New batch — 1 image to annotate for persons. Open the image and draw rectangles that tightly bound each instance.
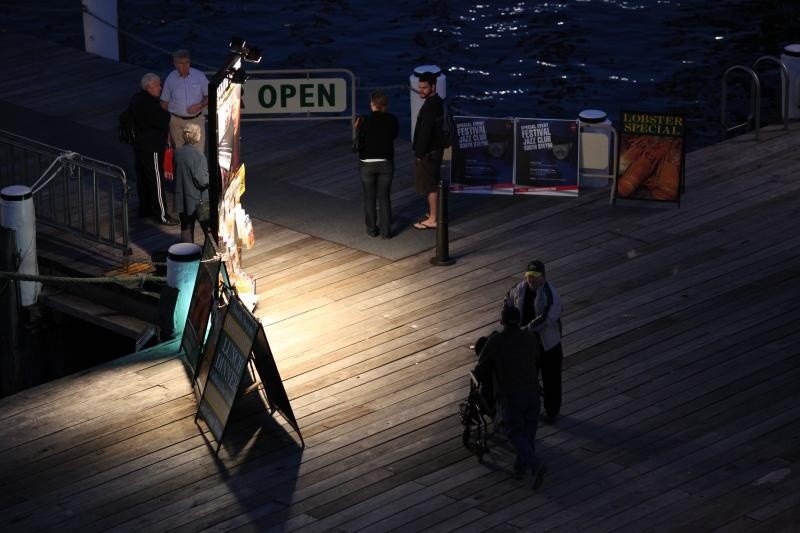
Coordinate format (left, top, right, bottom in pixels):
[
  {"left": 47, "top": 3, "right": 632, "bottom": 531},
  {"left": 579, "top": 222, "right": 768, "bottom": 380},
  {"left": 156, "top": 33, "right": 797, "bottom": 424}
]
[
  {"left": 411, "top": 71, "right": 453, "bottom": 230},
  {"left": 353, "top": 91, "right": 399, "bottom": 241},
  {"left": 471, "top": 260, "right": 565, "bottom": 452},
  {"left": 172, "top": 122, "right": 210, "bottom": 244},
  {"left": 119, "top": 49, "right": 210, "bottom": 226}
]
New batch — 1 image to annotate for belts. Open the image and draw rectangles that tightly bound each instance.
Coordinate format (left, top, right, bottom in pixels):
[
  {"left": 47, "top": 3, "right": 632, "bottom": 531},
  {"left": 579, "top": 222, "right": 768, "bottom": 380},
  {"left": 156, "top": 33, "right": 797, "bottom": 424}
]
[{"left": 171, "top": 111, "right": 203, "bottom": 120}]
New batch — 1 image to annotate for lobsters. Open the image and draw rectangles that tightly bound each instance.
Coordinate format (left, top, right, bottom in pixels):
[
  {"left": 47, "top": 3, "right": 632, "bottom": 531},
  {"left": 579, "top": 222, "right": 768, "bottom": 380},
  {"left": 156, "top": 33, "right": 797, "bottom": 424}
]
[{"left": 617, "top": 136, "right": 682, "bottom": 201}]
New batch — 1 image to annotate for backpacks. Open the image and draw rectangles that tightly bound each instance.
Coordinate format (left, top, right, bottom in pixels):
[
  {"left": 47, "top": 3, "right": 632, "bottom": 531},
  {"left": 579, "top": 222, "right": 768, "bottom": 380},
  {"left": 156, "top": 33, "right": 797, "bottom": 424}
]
[{"left": 431, "top": 102, "right": 456, "bottom": 149}]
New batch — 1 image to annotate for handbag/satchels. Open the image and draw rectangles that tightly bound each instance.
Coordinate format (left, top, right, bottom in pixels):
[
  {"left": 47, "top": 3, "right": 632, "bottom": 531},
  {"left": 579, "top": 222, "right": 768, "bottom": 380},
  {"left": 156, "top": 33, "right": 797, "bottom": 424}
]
[
  {"left": 117, "top": 100, "right": 135, "bottom": 145},
  {"left": 352, "top": 118, "right": 361, "bottom": 153},
  {"left": 197, "top": 201, "right": 210, "bottom": 222}
]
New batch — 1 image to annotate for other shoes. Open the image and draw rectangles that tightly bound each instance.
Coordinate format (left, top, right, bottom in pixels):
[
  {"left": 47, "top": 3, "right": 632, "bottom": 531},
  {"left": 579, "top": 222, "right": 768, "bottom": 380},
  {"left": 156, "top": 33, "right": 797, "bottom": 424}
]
[
  {"left": 512, "top": 478, "right": 526, "bottom": 488},
  {"left": 543, "top": 412, "right": 558, "bottom": 424},
  {"left": 529, "top": 459, "right": 547, "bottom": 489},
  {"left": 161, "top": 218, "right": 180, "bottom": 226}
]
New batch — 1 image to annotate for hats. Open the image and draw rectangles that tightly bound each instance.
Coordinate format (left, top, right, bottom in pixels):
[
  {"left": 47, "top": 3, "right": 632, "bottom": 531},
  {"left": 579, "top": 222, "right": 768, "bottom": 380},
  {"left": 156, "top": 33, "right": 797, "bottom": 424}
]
[{"left": 524, "top": 260, "right": 545, "bottom": 278}]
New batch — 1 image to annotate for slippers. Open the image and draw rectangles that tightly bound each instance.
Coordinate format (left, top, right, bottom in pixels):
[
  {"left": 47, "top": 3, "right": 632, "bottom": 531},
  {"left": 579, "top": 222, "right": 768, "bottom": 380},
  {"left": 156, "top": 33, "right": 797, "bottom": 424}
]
[
  {"left": 413, "top": 222, "right": 437, "bottom": 230},
  {"left": 424, "top": 212, "right": 430, "bottom": 218}
]
